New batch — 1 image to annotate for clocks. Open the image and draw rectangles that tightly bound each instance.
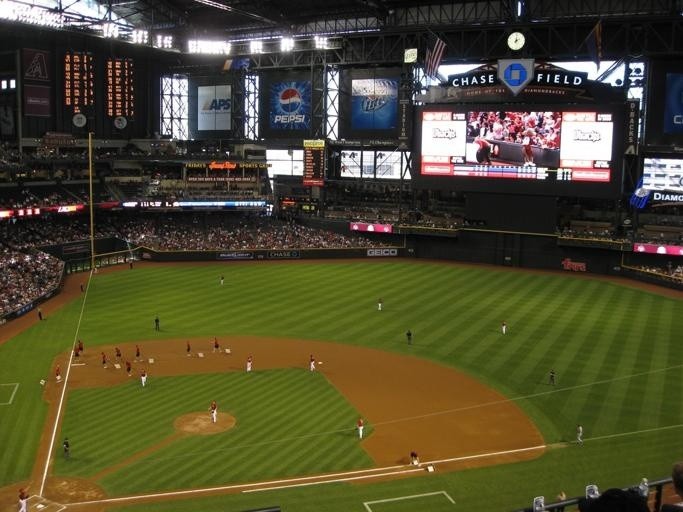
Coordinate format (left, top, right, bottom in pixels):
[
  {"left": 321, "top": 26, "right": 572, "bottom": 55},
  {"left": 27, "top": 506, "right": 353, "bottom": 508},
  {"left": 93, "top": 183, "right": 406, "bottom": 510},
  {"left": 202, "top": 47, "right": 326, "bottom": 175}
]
[{"left": 506, "top": 31, "right": 526, "bottom": 51}]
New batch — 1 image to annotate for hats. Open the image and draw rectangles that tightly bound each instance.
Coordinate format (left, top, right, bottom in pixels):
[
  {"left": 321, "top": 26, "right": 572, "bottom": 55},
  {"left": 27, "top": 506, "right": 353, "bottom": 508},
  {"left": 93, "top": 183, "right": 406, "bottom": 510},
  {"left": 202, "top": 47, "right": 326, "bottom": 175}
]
[{"left": 579, "top": 488, "right": 649, "bottom": 512}]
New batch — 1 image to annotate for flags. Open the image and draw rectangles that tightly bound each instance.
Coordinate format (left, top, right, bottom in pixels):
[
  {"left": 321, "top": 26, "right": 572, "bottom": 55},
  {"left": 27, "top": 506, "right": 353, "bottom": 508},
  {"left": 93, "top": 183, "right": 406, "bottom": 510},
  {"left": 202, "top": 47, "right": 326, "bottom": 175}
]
[
  {"left": 585, "top": 19, "right": 603, "bottom": 71},
  {"left": 425, "top": 29, "right": 447, "bottom": 81}
]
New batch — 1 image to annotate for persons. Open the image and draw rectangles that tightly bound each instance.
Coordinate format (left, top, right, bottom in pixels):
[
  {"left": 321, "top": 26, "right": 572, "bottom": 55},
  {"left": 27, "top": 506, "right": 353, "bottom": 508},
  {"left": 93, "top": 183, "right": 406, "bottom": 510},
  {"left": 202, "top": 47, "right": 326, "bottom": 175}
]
[
  {"left": 56, "top": 336, "right": 254, "bottom": 423},
  {"left": 576, "top": 424, "right": 583, "bottom": 445},
  {"left": 406, "top": 330, "right": 412, "bottom": 345},
  {"left": 500, "top": 319, "right": 506, "bottom": 334},
  {"left": 155, "top": 316, "right": 159, "bottom": 331},
  {"left": 523, "top": 462, "right": 683, "bottom": 512},
  {"left": 63, "top": 437, "right": 69, "bottom": 457},
  {"left": 549, "top": 369, "right": 556, "bottom": 385},
  {"left": 466, "top": 111, "right": 562, "bottom": 166},
  {"left": 19, "top": 488, "right": 29, "bottom": 512},
  {"left": 409, "top": 451, "right": 420, "bottom": 465},
  {"left": 357, "top": 416, "right": 363, "bottom": 439},
  {"left": 555, "top": 207, "right": 683, "bottom": 283},
  {"left": 310, "top": 354, "right": 315, "bottom": 371}
]
